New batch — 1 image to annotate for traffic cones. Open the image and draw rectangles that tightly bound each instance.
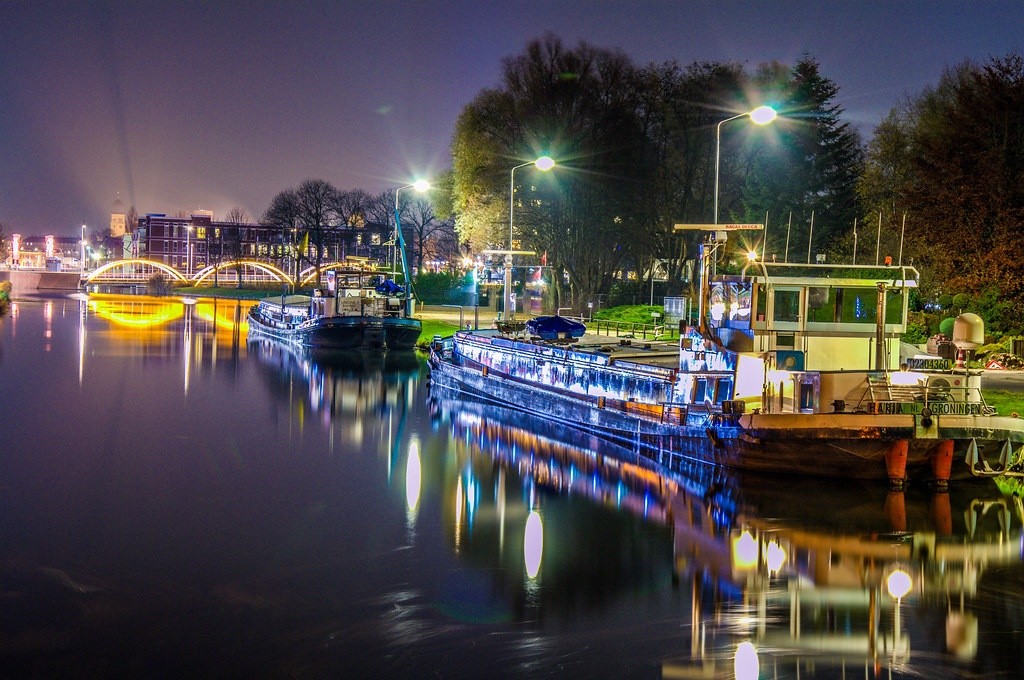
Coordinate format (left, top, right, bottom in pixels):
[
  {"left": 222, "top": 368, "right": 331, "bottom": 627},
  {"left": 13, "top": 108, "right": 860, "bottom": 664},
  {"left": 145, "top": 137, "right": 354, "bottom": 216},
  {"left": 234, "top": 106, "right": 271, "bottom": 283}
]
[{"left": 956, "top": 350, "right": 965, "bottom": 369}]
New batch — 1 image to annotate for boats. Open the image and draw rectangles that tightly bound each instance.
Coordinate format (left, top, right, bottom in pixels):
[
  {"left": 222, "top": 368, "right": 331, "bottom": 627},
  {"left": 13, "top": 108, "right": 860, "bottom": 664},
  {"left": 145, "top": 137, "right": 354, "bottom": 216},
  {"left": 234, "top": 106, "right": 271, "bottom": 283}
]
[
  {"left": 431, "top": 211, "right": 1024, "bottom": 492},
  {"left": 247, "top": 268, "right": 423, "bottom": 350}
]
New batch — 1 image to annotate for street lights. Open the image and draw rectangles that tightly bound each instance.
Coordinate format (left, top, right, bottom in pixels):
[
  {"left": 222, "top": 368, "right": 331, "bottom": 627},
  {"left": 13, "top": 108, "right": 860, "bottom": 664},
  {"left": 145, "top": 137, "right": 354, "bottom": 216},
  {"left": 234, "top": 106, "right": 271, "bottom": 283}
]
[
  {"left": 393, "top": 180, "right": 430, "bottom": 284},
  {"left": 81, "top": 224, "right": 86, "bottom": 242},
  {"left": 712, "top": 103, "right": 777, "bottom": 274},
  {"left": 186, "top": 227, "right": 193, "bottom": 278},
  {"left": 508, "top": 156, "right": 555, "bottom": 252}
]
